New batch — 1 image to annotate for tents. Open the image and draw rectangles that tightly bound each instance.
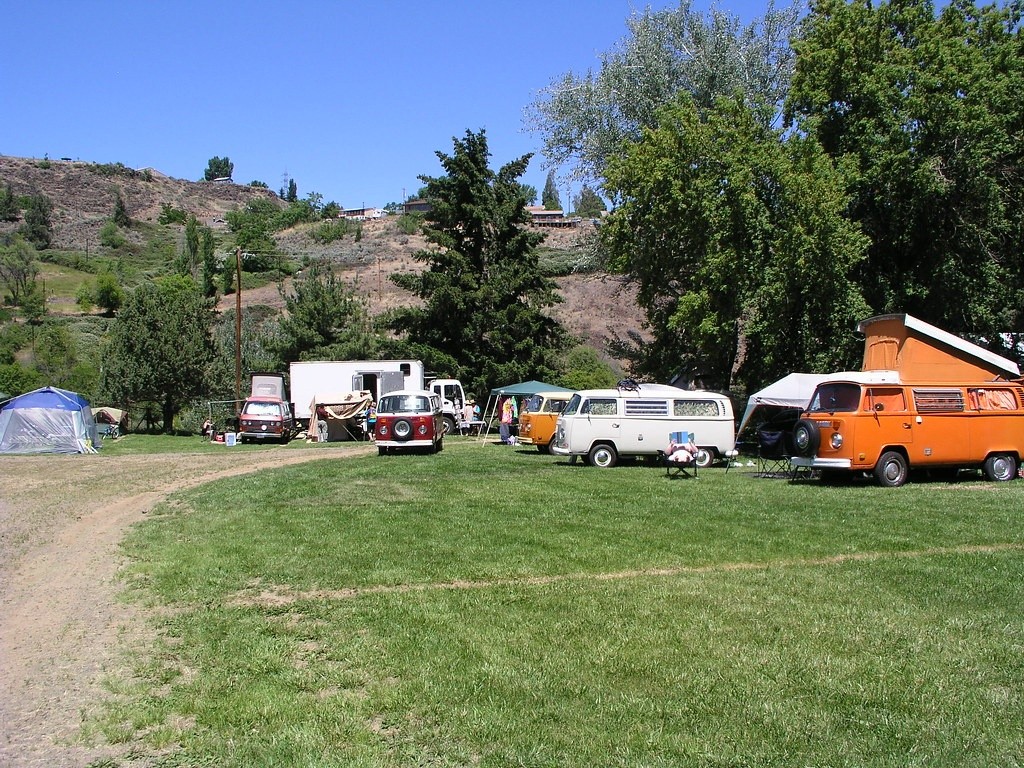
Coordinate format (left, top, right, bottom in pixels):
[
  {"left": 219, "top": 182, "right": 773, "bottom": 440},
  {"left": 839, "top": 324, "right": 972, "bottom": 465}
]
[
  {"left": 724, "top": 371, "right": 902, "bottom": 474},
  {"left": 474, "top": 379, "right": 578, "bottom": 448},
  {"left": 0, "top": 386, "right": 103, "bottom": 456}
]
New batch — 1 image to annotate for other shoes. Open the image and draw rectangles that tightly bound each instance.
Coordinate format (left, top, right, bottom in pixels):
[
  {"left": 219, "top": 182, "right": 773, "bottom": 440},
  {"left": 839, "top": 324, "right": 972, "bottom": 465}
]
[{"left": 369, "top": 438, "right": 374, "bottom": 443}]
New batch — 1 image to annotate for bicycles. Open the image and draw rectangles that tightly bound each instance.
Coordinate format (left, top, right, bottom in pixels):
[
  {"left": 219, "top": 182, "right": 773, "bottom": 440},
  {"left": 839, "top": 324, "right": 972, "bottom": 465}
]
[{"left": 102, "top": 422, "right": 120, "bottom": 440}]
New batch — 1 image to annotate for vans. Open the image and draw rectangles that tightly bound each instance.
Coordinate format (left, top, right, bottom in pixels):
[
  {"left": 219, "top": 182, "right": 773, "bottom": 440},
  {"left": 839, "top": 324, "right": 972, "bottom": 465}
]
[
  {"left": 374, "top": 390, "right": 448, "bottom": 455},
  {"left": 553, "top": 383, "right": 739, "bottom": 468},
  {"left": 517, "top": 390, "right": 572, "bottom": 454},
  {"left": 236, "top": 397, "right": 293, "bottom": 444},
  {"left": 788, "top": 311, "right": 1024, "bottom": 494}
]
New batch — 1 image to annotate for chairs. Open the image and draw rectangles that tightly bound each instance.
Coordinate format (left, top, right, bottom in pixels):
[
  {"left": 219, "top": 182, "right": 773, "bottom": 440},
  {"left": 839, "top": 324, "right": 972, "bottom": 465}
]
[
  {"left": 658, "top": 432, "right": 698, "bottom": 477},
  {"left": 753, "top": 428, "right": 792, "bottom": 478}
]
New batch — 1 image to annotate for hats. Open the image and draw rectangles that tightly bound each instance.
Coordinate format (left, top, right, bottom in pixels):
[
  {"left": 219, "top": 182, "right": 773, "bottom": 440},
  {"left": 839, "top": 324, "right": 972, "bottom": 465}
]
[
  {"left": 371, "top": 402, "right": 376, "bottom": 407},
  {"left": 464, "top": 400, "right": 472, "bottom": 404},
  {"left": 470, "top": 399, "right": 476, "bottom": 404}
]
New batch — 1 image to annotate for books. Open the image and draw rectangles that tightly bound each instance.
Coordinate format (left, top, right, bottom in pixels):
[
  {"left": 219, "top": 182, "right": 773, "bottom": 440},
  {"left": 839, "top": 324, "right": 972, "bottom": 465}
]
[{"left": 669, "top": 432, "right": 688, "bottom": 444}]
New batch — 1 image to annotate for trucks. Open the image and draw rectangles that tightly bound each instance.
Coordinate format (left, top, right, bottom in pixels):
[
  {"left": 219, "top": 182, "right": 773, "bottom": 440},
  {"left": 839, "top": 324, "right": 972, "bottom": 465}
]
[{"left": 290, "top": 359, "right": 470, "bottom": 437}]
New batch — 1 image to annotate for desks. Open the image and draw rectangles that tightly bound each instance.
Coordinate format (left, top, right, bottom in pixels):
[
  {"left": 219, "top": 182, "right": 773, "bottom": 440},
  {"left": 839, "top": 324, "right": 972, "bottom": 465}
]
[{"left": 459, "top": 420, "right": 486, "bottom": 436}]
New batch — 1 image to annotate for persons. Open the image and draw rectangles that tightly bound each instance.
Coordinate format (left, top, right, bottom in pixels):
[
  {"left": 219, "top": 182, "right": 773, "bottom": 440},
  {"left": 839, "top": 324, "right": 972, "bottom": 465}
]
[
  {"left": 665, "top": 439, "right": 699, "bottom": 463},
  {"left": 202, "top": 419, "right": 214, "bottom": 435},
  {"left": 365, "top": 402, "right": 378, "bottom": 442},
  {"left": 464, "top": 399, "right": 481, "bottom": 436},
  {"left": 316, "top": 403, "right": 328, "bottom": 442}
]
